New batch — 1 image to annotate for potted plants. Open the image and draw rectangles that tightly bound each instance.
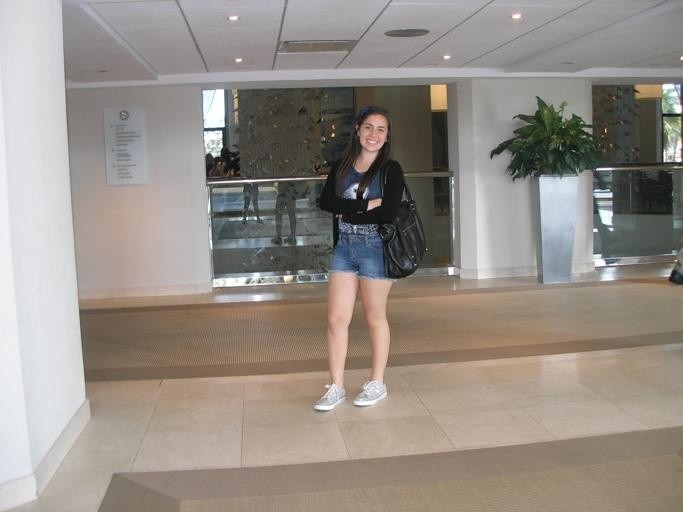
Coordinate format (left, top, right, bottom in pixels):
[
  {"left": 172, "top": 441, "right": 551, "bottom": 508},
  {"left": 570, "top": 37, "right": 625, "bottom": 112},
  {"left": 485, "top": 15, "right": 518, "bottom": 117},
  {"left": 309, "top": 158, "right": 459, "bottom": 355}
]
[{"left": 489, "top": 93, "right": 606, "bottom": 285}]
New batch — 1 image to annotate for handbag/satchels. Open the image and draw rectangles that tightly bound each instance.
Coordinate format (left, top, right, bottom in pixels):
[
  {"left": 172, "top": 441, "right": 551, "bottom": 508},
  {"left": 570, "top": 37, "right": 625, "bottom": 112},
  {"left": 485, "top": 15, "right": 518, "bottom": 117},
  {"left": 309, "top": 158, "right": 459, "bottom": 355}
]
[{"left": 375, "top": 158, "right": 427, "bottom": 279}]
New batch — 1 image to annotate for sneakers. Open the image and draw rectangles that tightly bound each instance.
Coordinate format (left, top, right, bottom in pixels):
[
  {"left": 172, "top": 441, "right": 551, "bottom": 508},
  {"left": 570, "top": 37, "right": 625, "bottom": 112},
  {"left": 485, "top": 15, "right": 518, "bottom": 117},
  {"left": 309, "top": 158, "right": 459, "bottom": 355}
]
[
  {"left": 257, "top": 218, "right": 263, "bottom": 223},
  {"left": 242, "top": 220, "right": 247, "bottom": 223},
  {"left": 284, "top": 238, "right": 296, "bottom": 245},
  {"left": 271, "top": 238, "right": 282, "bottom": 245},
  {"left": 313, "top": 382, "right": 346, "bottom": 411},
  {"left": 352, "top": 380, "right": 387, "bottom": 406}
]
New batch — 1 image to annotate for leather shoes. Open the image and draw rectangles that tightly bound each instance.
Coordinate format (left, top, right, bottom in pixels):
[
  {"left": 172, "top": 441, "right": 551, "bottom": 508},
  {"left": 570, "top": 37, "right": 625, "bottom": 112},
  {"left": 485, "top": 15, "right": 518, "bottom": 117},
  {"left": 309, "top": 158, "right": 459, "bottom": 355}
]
[{"left": 668, "top": 270, "right": 682, "bottom": 284}]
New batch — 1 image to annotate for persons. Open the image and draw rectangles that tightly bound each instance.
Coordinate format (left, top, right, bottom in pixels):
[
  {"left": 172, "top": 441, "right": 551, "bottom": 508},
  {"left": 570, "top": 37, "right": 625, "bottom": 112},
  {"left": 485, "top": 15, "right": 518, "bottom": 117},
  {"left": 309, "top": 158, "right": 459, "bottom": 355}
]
[
  {"left": 238, "top": 184, "right": 264, "bottom": 226},
  {"left": 312, "top": 105, "right": 404, "bottom": 413},
  {"left": 591, "top": 171, "right": 621, "bottom": 247},
  {"left": 269, "top": 180, "right": 301, "bottom": 247}
]
